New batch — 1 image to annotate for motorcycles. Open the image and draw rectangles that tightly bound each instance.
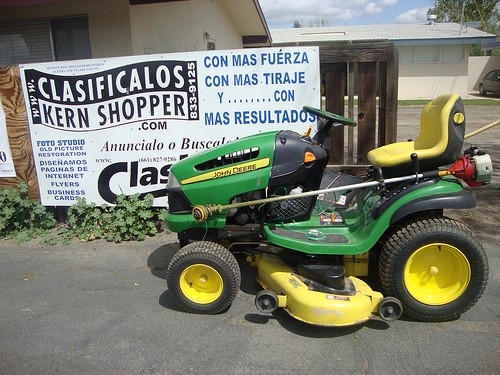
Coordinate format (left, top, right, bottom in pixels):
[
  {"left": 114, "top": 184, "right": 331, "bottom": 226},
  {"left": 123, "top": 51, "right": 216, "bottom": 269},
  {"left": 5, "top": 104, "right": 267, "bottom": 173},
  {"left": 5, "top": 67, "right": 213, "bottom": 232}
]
[{"left": 162, "top": 94, "right": 489, "bottom": 330}]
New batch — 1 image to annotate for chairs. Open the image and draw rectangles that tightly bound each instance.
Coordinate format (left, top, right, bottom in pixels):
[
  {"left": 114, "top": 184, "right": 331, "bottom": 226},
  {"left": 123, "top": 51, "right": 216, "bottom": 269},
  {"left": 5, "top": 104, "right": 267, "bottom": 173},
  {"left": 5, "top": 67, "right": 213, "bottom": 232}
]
[{"left": 366, "top": 93, "right": 466, "bottom": 172}]
